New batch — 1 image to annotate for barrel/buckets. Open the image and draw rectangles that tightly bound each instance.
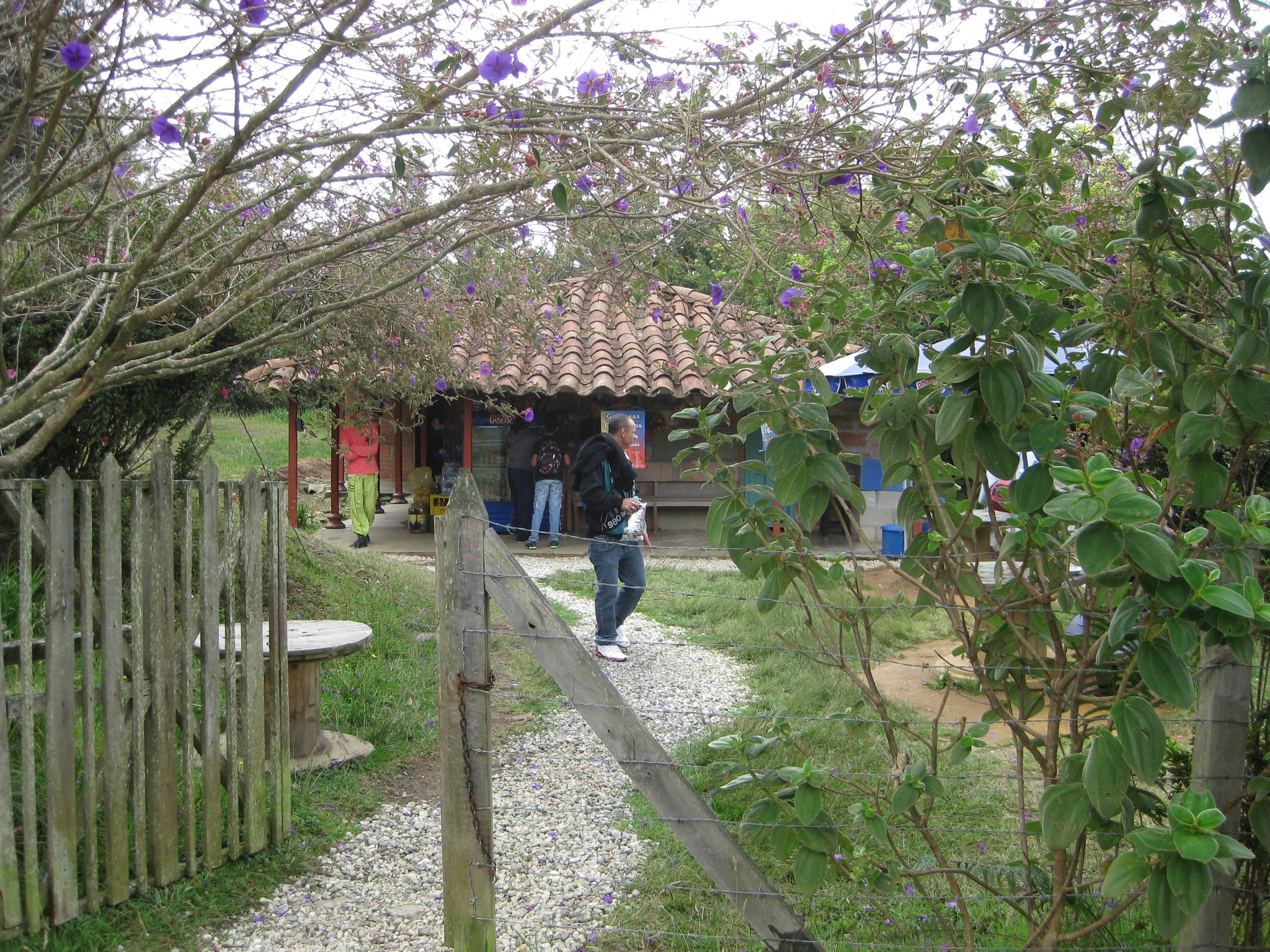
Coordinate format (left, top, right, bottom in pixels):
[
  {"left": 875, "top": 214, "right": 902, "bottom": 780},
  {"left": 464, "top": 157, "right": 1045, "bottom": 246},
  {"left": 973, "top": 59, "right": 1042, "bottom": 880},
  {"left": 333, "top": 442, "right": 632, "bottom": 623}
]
[{"left": 880, "top": 524, "right": 905, "bottom": 558}]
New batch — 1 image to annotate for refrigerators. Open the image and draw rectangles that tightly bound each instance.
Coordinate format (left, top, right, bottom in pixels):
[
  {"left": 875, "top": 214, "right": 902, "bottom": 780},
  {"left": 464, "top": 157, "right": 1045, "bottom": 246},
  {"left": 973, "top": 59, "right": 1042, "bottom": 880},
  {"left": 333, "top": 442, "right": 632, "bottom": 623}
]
[{"left": 466, "top": 397, "right": 564, "bottom": 535}]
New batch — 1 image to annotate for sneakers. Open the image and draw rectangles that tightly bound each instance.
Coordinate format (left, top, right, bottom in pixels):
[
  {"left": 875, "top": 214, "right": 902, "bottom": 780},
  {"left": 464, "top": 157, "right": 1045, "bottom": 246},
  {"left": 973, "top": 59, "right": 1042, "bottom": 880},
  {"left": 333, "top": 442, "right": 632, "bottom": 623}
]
[
  {"left": 615, "top": 628, "right": 631, "bottom": 647},
  {"left": 550, "top": 540, "right": 560, "bottom": 548},
  {"left": 525, "top": 541, "right": 537, "bottom": 549},
  {"left": 596, "top": 642, "right": 627, "bottom": 662}
]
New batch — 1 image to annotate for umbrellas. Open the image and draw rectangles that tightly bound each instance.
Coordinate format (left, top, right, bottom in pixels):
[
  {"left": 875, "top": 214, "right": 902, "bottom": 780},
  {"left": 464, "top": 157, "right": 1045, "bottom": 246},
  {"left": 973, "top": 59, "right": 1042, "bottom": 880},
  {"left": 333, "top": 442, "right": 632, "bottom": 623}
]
[{"left": 762, "top": 332, "right": 1135, "bottom": 480}]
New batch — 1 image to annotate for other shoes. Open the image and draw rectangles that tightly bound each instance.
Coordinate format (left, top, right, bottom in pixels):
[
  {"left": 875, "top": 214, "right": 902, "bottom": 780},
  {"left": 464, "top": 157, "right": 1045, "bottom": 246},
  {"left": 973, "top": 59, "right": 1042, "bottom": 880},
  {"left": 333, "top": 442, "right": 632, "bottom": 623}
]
[
  {"left": 508, "top": 530, "right": 518, "bottom": 537},
  {"left": 516, "top": 536, "right": 530, "bottom": 543},
  {"left": 364, "top": 533, "right": 370, "bottom": 542},
  {"left": 349, "top": 536, "right": 367, "bottom": 549}
]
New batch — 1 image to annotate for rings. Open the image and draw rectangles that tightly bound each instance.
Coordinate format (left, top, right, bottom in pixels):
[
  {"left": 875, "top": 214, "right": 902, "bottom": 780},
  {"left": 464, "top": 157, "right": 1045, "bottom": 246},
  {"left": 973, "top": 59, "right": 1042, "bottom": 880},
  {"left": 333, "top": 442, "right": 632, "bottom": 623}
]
[{"left": 632, "top": 506, "right": 635, "bottom": 510}]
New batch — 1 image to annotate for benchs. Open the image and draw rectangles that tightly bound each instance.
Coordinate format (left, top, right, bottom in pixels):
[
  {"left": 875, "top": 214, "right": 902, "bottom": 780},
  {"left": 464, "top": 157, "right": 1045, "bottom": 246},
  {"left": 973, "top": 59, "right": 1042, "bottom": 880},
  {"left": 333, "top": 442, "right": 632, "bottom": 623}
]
[{"left": 571, "top": 460, "right": 740, "bottom": 537}]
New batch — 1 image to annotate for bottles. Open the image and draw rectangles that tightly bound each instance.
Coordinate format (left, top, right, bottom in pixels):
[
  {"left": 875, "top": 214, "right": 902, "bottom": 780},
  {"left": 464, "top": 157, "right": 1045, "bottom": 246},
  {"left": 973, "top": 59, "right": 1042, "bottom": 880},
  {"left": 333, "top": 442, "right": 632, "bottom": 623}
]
[
  {"left": 432, "top": 458, "right": 462, "bottom": 507},
  {"left": 633, "top": 496, "right": 647, "bottom": 531},
  {"left": 470, "top": 427, "right": 511, "bottom": 501},
  {"left": 407, "top": 487, "right": 431, "bottom": 534}
]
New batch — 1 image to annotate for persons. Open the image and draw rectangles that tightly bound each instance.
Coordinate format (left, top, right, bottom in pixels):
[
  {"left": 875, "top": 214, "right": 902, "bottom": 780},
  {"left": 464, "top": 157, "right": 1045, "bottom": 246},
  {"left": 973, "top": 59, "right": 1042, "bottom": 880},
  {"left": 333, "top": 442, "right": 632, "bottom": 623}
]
[
  {"left": 339, "top": 401, "right": 382, "bottom": 549},
  {"left": 502, "top": 416, "right": 603, "bottom": 549},
  {"left": 568, "top": 413, "right": 654, "bottom": 661},
  {"left": 425, "top": 412, "right": 463, "bottom": 479}
]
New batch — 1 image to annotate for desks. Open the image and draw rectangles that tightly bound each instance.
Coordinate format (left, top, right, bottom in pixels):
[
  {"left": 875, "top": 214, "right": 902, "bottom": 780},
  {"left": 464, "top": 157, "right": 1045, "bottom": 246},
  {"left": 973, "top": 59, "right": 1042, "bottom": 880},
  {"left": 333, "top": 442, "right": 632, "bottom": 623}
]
[
  {"left": 191, "top": 619, "right": 377, "bottom": 773},
  {"left": 919, "top": 509, "right": 1016, "bottom": 561}
]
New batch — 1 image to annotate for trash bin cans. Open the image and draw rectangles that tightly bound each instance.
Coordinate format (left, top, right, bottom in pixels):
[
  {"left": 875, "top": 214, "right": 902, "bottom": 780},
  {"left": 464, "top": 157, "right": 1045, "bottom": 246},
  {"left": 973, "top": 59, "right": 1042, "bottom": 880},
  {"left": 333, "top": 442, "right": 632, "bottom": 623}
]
[{"left": 881, "top": 524, "right": 904, "bottom": 558}]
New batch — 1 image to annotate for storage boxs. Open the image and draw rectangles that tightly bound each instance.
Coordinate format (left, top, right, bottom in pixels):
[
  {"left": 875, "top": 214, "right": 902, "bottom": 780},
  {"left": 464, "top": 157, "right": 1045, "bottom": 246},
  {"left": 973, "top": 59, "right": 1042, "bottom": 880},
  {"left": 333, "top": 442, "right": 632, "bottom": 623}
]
[{"left": 430, "top": 494, "right": 451, "bottom": 516}]
